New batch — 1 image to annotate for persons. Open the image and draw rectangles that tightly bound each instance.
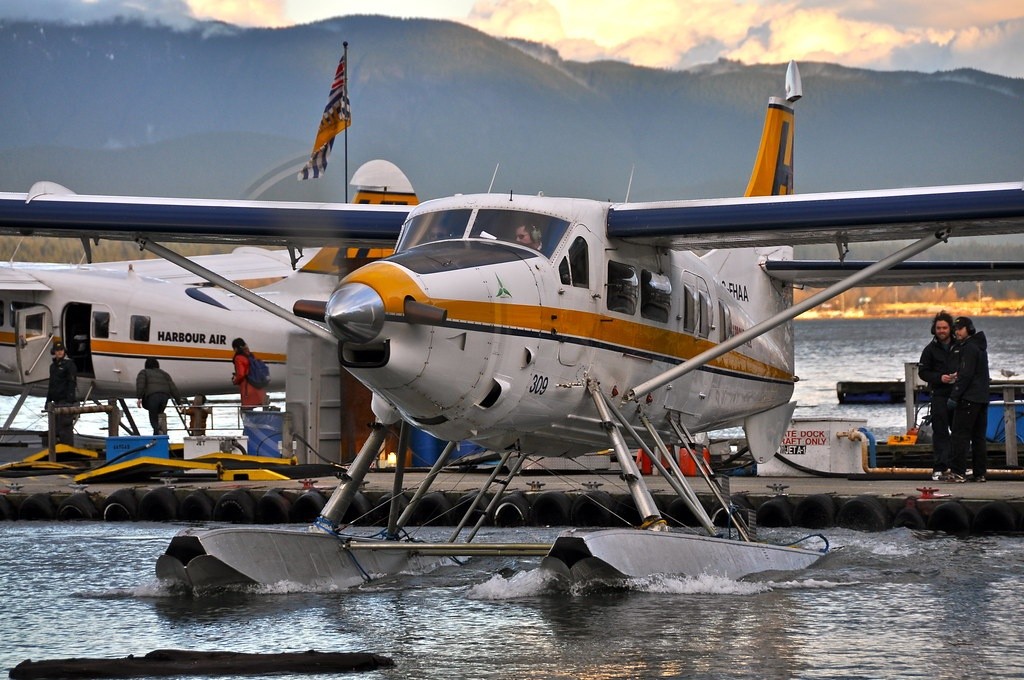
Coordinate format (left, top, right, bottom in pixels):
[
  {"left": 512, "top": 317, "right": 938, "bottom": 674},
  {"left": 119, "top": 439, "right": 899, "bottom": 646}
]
[
  {"left": 918, "top": 309, "right": 963, "bottom": 480},
  {"left": 938, "top": 316, "right": 989, "bottom": 483},
  {"left": 231, "top": 337, "right": 268, "bottom": 415},
  {"left": 427, "top": 221, "right": 452, "bottom": 241},
  {"left": 136, "top": 358, "right": 181, "bottom": 435},
  {"left": 184, "top": 394, "right": 212, "bottom": 437},
  {"left": 516, "top": 219, "right": 546, "bottom": 254},
  {"left": 45, "top": 342, "right": 78, "bottom": 446}
]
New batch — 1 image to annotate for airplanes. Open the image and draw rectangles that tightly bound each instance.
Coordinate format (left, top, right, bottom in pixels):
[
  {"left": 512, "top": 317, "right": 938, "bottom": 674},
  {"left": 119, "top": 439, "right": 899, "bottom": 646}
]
[{"left": 0, "top": 59, "right": 1023, "bottom": 592}]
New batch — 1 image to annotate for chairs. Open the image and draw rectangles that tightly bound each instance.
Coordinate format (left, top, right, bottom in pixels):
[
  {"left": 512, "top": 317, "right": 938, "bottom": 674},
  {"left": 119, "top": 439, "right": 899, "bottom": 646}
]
[
  {"left": 609, "top": 293, "right": 636, "bottom": 315},
  {"left": 643, "top": 303, "right": 669, "bottom": 321}
]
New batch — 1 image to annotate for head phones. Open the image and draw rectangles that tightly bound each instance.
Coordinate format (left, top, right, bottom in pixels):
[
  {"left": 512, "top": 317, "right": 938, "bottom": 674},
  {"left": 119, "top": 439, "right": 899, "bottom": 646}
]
[
  {"left": 529, "top": 224, "right": 543, "bottom": 242},
  {"left": 965, "top": 317, "right": 975, "bottom": 335},
  {"left": 929, "top": 318, "right": 954, "bottom": 334}
]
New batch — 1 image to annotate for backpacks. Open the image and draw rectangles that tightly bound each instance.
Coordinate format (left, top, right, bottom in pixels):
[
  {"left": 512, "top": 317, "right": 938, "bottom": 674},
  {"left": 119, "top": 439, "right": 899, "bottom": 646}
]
[{"left": 237, "top": 352, "right": 271, "bottom": 387}]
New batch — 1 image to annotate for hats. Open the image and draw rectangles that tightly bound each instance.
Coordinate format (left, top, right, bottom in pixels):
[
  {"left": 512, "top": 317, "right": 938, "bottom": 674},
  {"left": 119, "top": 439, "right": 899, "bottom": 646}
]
[
  {"left": 952, "top": 316, "right": 972, "bottom": 329},
  {"left": 53, "top": 342, "right": 64, "bottom": 350}
]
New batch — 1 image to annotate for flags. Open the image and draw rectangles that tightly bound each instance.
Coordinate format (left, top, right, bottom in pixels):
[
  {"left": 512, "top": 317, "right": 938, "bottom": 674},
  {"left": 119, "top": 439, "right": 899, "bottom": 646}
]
[{"left": 296, "top": 52, "right": 351, "bottom": 181}]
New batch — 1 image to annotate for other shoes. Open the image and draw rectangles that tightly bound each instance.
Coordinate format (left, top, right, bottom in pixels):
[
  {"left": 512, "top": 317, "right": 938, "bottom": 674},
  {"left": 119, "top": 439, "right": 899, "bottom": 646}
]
[
  {"left": 931, "top": 471, "right": 942, "bottom": 481},
  {"left": 938, "top": 472, "right": 966, "bottom": 482},
  {"left": 969, "top": 474, "right": 985, "bottom": 482}
]
